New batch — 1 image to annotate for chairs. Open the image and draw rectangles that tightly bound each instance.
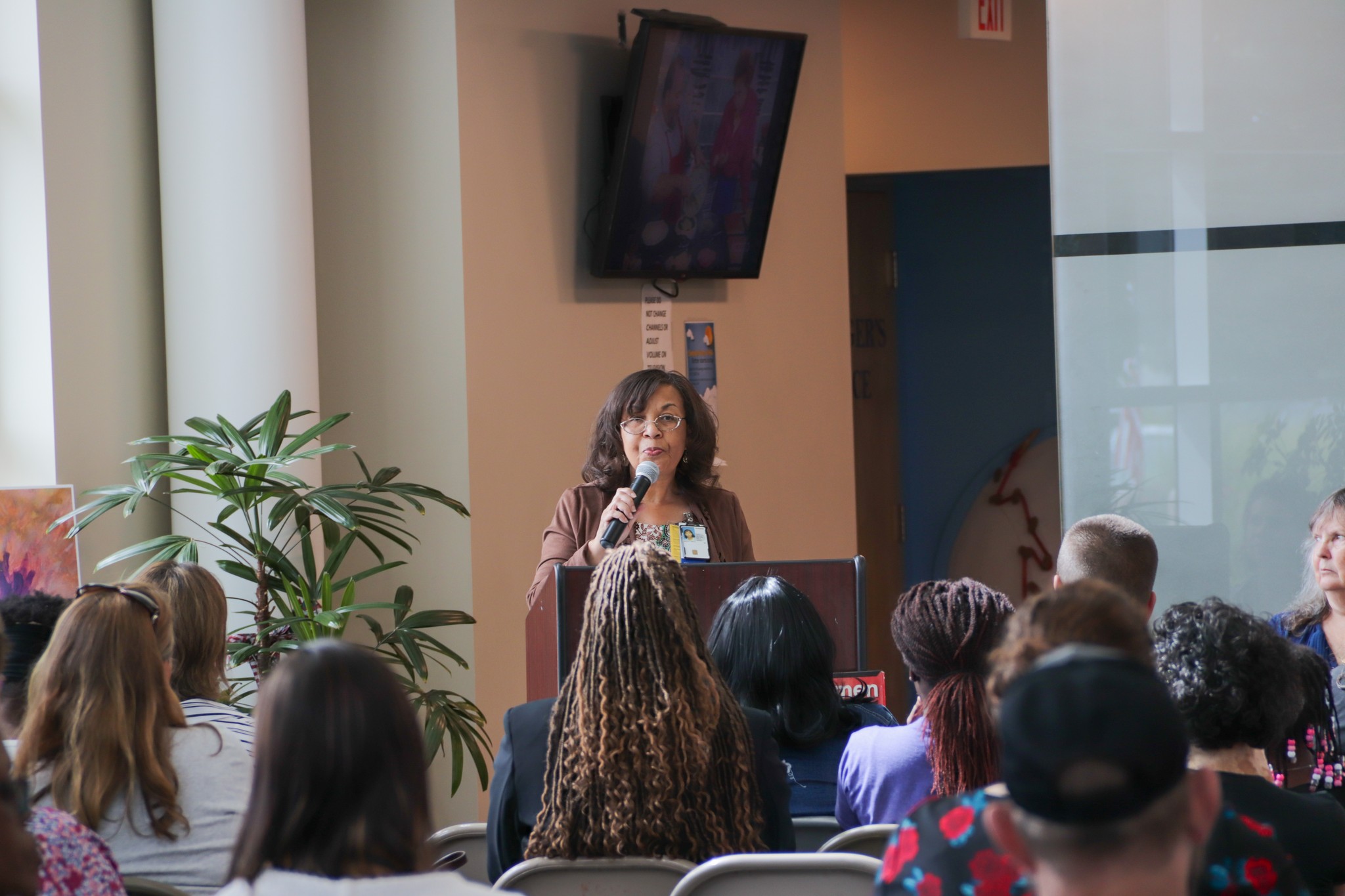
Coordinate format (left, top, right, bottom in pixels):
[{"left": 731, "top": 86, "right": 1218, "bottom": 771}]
[{"left": 423, "top": 813, "right": 905, "bottom": 896}]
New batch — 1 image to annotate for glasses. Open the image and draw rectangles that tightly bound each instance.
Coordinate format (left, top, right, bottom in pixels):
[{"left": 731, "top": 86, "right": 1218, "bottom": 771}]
[
  {"left": 75, "top": 582, "right": 161, "bottom": 625},
  {"left": 619, "top": 415, "right": 686, "bottom": 435}
]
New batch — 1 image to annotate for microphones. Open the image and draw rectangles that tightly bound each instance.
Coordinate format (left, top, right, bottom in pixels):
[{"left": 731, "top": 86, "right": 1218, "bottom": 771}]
[{"left": 600, "top": 461, "right": 659, "bottom": 549}]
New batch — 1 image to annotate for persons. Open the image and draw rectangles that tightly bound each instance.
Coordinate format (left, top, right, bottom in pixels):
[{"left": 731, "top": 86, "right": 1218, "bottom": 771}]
[
  {"left": 12, "top": 580, "right": 254, "bottom": 896},
  {"left": 706, "top": 576, "right": 900, "bottom": 817},
  {"left": 1265, "top": 642, "right": 1345, "bottom": 807},
  {"left": 1150, "top": 594, "right": 1345, "bottom": 896},
  {"left": 1266, "top": 487, "right": 1345, "bottom": 754},
  {"left": 526, "top": 370, "right": 756, "bottom": 614},
  {"left": 871, "top": 574, "right": 1308, "bottom": 896},
  {"left": 1053, "top": 513, "right": 1160, "bottom": 623},
  {"left": 488, "top": 540, "right": 797, "bottom": 883},
  {"left": 134, "top": 561, "right": 259, "bottom": 760},
  {"left": 0, "top": 587, "right": 129, "bottom": 896},
  {"left": 984, "top": 654, "right": 1221, "bottom": 896},
  {"left": 831, "top": 577, "right": 1018, "bottom": 828},
  {"left": 216, "top": 639, "right": 529, "bottom": 896}
]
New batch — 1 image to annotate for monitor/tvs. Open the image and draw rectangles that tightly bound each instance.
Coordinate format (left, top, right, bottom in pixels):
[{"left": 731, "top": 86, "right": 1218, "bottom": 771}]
[{"left": 588, "top": 10, "right": 808, "bottom": 280}]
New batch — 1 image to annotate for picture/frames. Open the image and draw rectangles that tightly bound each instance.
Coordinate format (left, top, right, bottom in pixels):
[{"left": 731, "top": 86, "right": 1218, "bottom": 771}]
[{"left": 0, "top": 483, "right": 83, "bottom": 603}]
